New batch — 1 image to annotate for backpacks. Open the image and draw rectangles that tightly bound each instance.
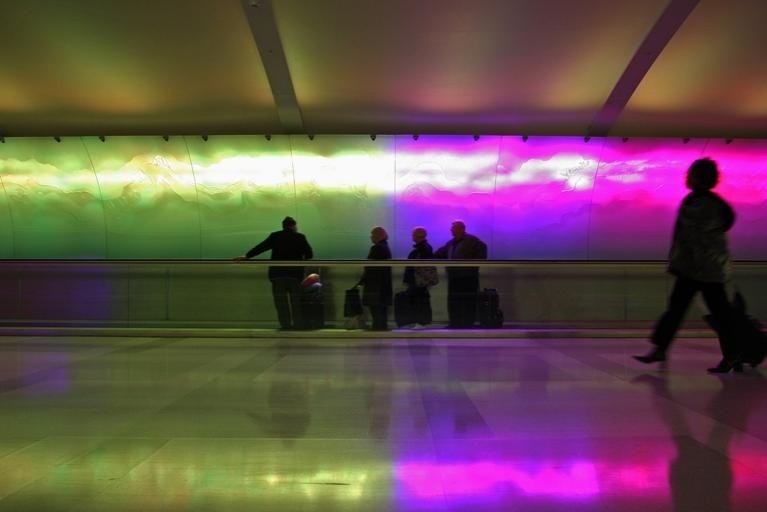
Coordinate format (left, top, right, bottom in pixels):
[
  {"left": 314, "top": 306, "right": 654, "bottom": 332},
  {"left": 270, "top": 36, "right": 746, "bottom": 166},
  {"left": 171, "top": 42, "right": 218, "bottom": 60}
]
[{"left": 701, "top": 290, "right": 766, "bottom": 368}]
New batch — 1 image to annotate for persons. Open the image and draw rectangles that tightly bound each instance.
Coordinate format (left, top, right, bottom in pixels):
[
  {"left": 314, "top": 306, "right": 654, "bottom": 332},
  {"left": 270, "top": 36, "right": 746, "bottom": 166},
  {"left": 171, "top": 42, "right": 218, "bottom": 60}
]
[
  {"left": 629, "top": 155, "right": 745, "bottom": 375},
  {"left": 355, "top": 224, "right": 394, "bottom": 332},
  {"left": 233, "top": 214, "right": 314, "bottom": 332},
  {"left": 401, "top": 225, "right": 434, "bottom": 331},
  {"left": 431, "top": 220, "right": 488, "bottom": 328}
]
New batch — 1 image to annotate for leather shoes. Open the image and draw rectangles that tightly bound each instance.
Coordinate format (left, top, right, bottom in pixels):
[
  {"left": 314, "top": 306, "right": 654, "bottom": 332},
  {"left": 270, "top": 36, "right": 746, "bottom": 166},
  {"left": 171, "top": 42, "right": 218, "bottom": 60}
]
[
  {"left": 707, "top": 352, "right": 742, "bottom": 374},
  {"left": 632, "top": 345, "right": 666, "bottom": 364}
]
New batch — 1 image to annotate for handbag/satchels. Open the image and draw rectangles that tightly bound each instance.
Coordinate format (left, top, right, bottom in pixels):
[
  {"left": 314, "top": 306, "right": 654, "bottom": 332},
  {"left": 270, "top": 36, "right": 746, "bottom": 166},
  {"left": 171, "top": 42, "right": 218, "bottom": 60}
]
[
  {"left": 478, "top": 286, "right": 503, "bottom": 327},
  {"left": 413, "top": 266, "right": 439, "bottom": 289},
  {"left": 394, "top": 289, "right": 415, "bottom": 327},
  {"left": 344, "top": 287, "right": 363, "bottom": 317}
]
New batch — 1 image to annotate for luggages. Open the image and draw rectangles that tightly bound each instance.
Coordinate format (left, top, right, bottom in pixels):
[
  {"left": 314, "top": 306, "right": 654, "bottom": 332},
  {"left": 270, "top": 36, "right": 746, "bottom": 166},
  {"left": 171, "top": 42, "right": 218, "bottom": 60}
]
[{"left": 296, "top": 292, "right": 324, "bottom": 329}]
